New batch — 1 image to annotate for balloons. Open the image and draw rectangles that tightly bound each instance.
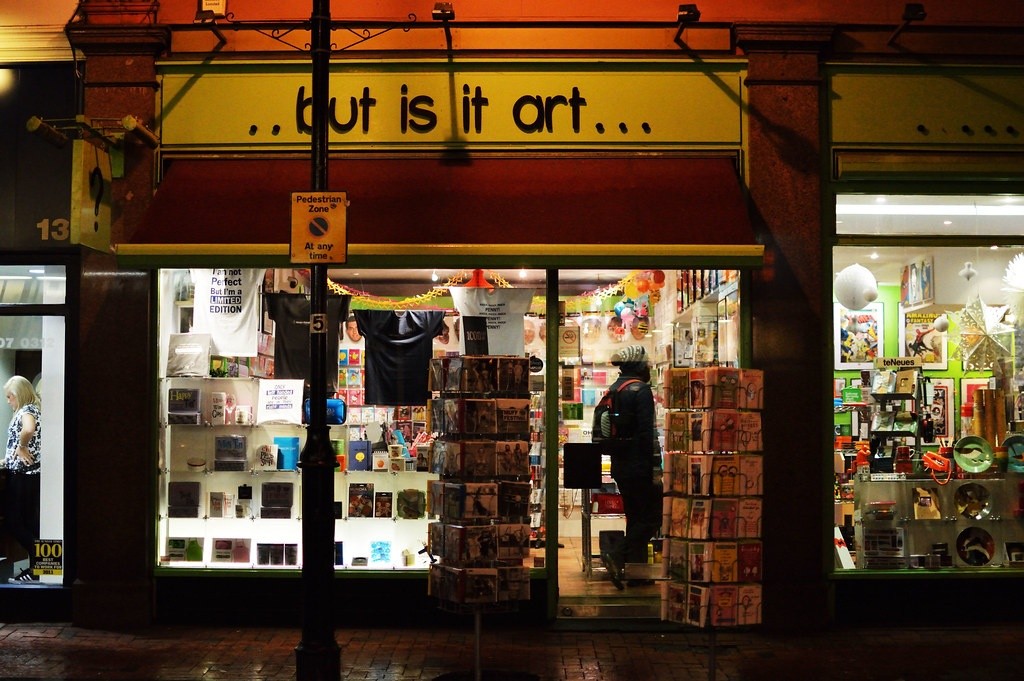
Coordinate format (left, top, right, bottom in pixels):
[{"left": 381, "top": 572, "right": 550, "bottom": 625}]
[{"left": 614, "top": 271, "right": 666, "bottom": 320}]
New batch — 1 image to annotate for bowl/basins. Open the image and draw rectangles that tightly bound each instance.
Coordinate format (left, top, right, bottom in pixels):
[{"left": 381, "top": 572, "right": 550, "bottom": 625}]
[
  {"left": 187, "top": 458, "right": 206, "bottom": 472},
  {"left": 870, "top": 502, "right": 897, "bottom": 510}
]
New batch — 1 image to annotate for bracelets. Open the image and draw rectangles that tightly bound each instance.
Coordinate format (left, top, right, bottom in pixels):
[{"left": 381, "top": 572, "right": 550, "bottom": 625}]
[{"left": 18, "top": 444, "right": 25, "bottom": 448}]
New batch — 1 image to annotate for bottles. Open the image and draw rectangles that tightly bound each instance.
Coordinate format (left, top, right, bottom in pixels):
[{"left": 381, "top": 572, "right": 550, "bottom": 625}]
[{"left": 562, "top": 401, "right": 582, "bottom": 421}]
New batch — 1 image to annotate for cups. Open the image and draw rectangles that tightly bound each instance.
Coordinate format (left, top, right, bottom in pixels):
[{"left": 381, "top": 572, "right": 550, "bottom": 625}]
[
  {"left": 222, "top": 494, "right": 235, "bottom": 518},
  {"left": 912, "top": 459, "right": 929, "bottom": 475},
  {"left": 993, "top": 446, "right": 1008, "bottom": 473},
  {"left": 897, "top": 446, "right": 915, "bottom": 459},
  {"left": 212, "top": 392, "right": 225, "bottom": 425},
  {"left": 834, "top": 424, "right": 850, "bottom": 436},
  {"left": 331, "top": 439, "right": 344, "bottom": 454},
  {"left": 285, "top": 544, "right": 298, "bottom": 566},
  {"left": 271, "top": 544, "right": 284, "bottom": 565},
  {"left": 406, "top": 461, "right": 414, "bottom": 471},
  {"left": 238, "top": 484, "right": 252, "bottom": 518},
  {"left": 257, "top": 543, "right": 269, "bottom": 565},
  {"left": 235, "top": 405, "right": 253, "bottom": 425},
  {"left": 387, "top": 445, "right": 407, "bottom": 458}
]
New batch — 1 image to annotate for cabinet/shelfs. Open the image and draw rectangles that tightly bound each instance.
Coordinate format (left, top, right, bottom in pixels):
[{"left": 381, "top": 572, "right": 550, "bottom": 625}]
[
  {"left": 866, "top": 366, "right": 923, "bottom": 473},
  {"left": 858, "top": 479, "right": 1006, "bottom": 571},
  {"left": 164, "top": 375, "right": 435, "bottom": 570},
  {"left": 580, "top": 473, "right": 627, "bottom": 580}
]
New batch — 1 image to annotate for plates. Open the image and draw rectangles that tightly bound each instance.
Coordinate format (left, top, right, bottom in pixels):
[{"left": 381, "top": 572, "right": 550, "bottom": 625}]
[
  {"left": 1001, "top": 434, "right": 1024, "bottom": 473},
  {"left": 953, "top": 435, "right": 994, "bottom": 473},
  {"left": 956, "top": 527, "right": 994, "bottom": 566},
  {"left": 954, "top": 483, "right": 993, "bottom": 521}
]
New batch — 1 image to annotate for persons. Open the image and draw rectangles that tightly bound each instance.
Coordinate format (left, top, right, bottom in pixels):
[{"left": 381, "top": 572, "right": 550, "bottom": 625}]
[
  {"left": 607, "top": 317, "right": 626, "bottom": 342},
  {"left": 4, "top": 376, "right": 42, "bottom": 584},
  {"left": 345, "top": 316, "right": 362, "bottom": 341},
  {"left": 436, "top": 318, "right": 460, "bottom": 344},
  {"left": 582, "top": 319, "right": 600, "bottom": 343},
  {"left": 525, "top": 320, "right": 579, "bottom": 345},
  {"left": 600, "top": 346, "right": 664, "bottom": 590},
  {"left": 631, "top": 317, "right": 648, "bottom": 340}
]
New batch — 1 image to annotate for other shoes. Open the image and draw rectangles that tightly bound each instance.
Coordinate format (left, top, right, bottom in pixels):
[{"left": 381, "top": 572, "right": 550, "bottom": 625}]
[
  {"left": 601, "top": 553, "right": 625, "bottom": 590},
  {"left": 627, "top": 576, "right": 654, "bottom": 587}
]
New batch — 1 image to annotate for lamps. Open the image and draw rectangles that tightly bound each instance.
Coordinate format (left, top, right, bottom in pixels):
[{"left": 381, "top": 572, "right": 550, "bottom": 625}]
[
  {"left": 672, "top": 3, "right": 701, "bottom": 43},
  {"left": 888, "top": 3, "right": 927, "bottom": 47},
  {"left": 194, "top": 10, "right": 228, "bottom": 44},
  {"left": 431, "top": 2, "right": 455, "bottom": 39}
]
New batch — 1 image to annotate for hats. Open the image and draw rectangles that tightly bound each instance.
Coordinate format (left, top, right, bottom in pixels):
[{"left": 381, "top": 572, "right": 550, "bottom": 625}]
[{"left": 611, "top": 344, "right": 649, "bottom": 377}]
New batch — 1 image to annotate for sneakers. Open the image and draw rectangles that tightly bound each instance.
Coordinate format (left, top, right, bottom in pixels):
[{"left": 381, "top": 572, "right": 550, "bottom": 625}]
[{"left": 8, "top": 568, "right": 41, "bottom": 585}]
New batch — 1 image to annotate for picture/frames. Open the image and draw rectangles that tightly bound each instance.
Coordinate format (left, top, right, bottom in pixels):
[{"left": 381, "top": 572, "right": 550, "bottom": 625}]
[{"left": 833, "top": 300, "right": 1007, "bottom": 450}]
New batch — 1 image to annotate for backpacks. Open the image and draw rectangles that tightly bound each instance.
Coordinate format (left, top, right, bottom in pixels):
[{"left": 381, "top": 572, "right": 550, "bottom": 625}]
[{"left": 590, "top": 379, "right": 651, "bottom": 454}]
